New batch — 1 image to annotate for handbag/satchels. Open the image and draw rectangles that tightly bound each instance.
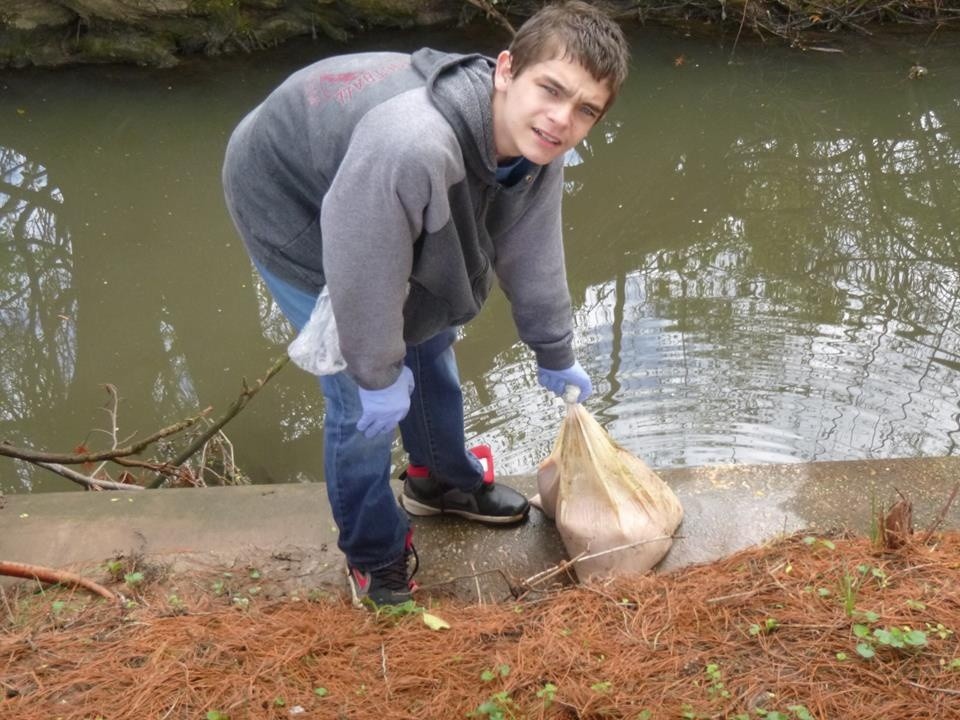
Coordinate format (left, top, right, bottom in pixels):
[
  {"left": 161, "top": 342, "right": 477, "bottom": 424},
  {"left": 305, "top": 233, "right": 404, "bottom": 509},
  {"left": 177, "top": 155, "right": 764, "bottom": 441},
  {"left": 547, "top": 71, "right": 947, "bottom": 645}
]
[{"left": 536, "top": 383, "right": 685, "bottom": 585}]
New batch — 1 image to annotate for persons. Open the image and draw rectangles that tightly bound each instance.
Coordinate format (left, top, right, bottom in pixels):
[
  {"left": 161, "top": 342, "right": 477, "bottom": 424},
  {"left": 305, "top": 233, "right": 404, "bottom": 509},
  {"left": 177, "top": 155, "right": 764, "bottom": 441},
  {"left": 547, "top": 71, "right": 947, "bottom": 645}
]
[{"left": 222, "top": 0, "right": 630, "bottom": 614}]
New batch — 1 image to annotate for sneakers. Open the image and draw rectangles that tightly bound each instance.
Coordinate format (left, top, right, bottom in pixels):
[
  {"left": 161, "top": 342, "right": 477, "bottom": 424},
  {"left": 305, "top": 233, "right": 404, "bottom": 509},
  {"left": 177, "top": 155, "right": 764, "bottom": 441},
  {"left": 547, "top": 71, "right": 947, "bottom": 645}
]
[
  {"left": 344, "top": 521, "right": 420, "bottom": 614},
  {"left": 399, "top": 444, "right": 531, "bottom": 527}
]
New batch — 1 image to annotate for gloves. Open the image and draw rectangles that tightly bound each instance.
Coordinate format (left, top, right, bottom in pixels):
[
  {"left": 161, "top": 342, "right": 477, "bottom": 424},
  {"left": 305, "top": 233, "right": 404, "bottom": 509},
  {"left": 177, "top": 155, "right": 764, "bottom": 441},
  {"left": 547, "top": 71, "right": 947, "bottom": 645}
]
[
  {"left": 355, "top": 364, "right": 416, "bottom": 442},
  {"left": 537, "top": 356, "right": 593, "bottom": 404}
]
